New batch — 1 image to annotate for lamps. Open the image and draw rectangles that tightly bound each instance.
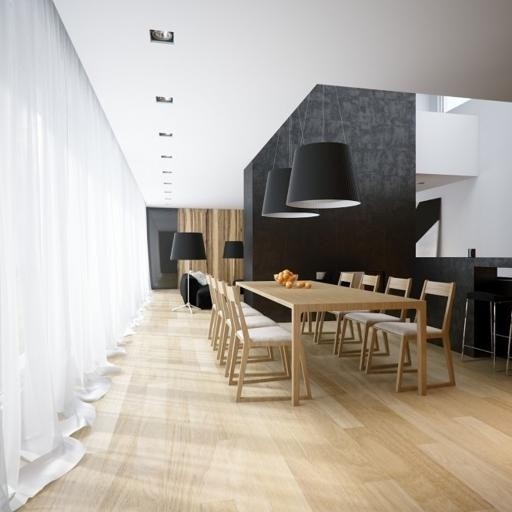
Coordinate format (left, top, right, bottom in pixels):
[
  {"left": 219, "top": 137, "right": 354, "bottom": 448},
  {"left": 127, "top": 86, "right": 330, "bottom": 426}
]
[
  {"left": 261, "top": 168, "right": 320, "bottom": 219},
  {"left": 285, "top": 142, "right": 362, "bottom": 210},
  {"left": 170, "top": 232, "right": 207, "bottom": 314},
  {"left": 223, "top": 240, "right": 245, "bottom": 259}
]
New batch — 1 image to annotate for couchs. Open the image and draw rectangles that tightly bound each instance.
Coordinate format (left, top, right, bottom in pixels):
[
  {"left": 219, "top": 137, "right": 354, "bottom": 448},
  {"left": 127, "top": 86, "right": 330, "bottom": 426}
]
[{"left": 180, "top": 271, "right": 213, "bottom": 309}]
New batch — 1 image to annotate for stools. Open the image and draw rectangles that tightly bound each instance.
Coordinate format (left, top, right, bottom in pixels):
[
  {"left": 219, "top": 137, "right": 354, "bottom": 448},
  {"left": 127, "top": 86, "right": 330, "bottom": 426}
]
[{"left": 460, "top": 291, "right": 507, "bottom": 369}]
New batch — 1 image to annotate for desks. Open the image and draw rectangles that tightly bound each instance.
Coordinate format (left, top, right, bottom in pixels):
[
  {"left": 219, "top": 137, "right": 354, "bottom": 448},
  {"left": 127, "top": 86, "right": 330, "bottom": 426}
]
[
  {"left": 413, "top": 256, "right": 512, "bottom": 358},
  {"left": 236, "top": 281, "right": 427, "bottom": 407}
]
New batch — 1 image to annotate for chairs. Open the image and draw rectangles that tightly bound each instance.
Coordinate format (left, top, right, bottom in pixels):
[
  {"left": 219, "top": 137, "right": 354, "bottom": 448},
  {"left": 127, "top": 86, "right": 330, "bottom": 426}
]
[
  {"left": 208, "top": 273, "right": 270, "bottom": 359},
  {"left": 300, "top": 271, "right": 356, "bottom": 344},
  {"left": 338, "top": 277, "right": 414, "bottom": 372},
  {"left": 364, "top": 281, "right": 457, "bottom": 392},
  {"left": 216, "top": 281, "right": 285, "bottom": 378},
  {"left": 228, "top": 282, "right": 312, "bottom": 403},
  {"left": 317, "top": 273, "right": 381, "bottom": 355}
]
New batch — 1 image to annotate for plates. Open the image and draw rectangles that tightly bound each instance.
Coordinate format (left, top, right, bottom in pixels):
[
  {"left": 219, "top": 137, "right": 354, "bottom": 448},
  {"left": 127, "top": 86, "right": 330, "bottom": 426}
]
[{"left": 273, "top": 275, "right": 298, "bottom": 287}]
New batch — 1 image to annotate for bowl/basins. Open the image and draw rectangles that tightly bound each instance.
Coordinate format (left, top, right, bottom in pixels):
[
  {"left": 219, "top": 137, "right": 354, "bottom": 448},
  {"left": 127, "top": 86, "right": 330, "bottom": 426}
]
[{"left": 316, "top": 272, "right": 326, "bottom": 279}]
